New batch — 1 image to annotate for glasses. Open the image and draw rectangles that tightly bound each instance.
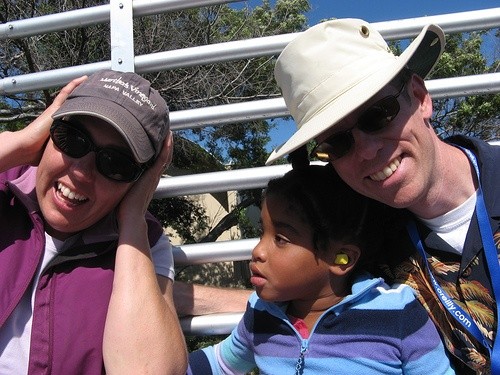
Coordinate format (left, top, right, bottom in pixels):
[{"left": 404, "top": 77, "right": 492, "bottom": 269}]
[
  {"left": 309, "top": 78, "right": 408, "bottom": 163},
  {"left": 49, "top": 113, "right": 141, "bottom": 183}
]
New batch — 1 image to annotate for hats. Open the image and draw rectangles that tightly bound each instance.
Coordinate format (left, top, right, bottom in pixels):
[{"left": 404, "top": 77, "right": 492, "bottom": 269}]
[
  {"left": 264, "top": 18, "right": 446, "bottom": 166},
  {"left": 51, "top": 70, "right": 170, "bottom": 163}
]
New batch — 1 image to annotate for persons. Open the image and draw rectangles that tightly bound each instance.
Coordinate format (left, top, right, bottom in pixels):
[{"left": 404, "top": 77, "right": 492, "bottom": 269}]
[
  {"left": 0, "top": 68, "right": 189, "bottom": 374},
  {"left": 183, "top": 165, "right": 458, "bottom": 375},
  {"left": 265, "top": 17, "right": 500, "bottom": 375}
]
